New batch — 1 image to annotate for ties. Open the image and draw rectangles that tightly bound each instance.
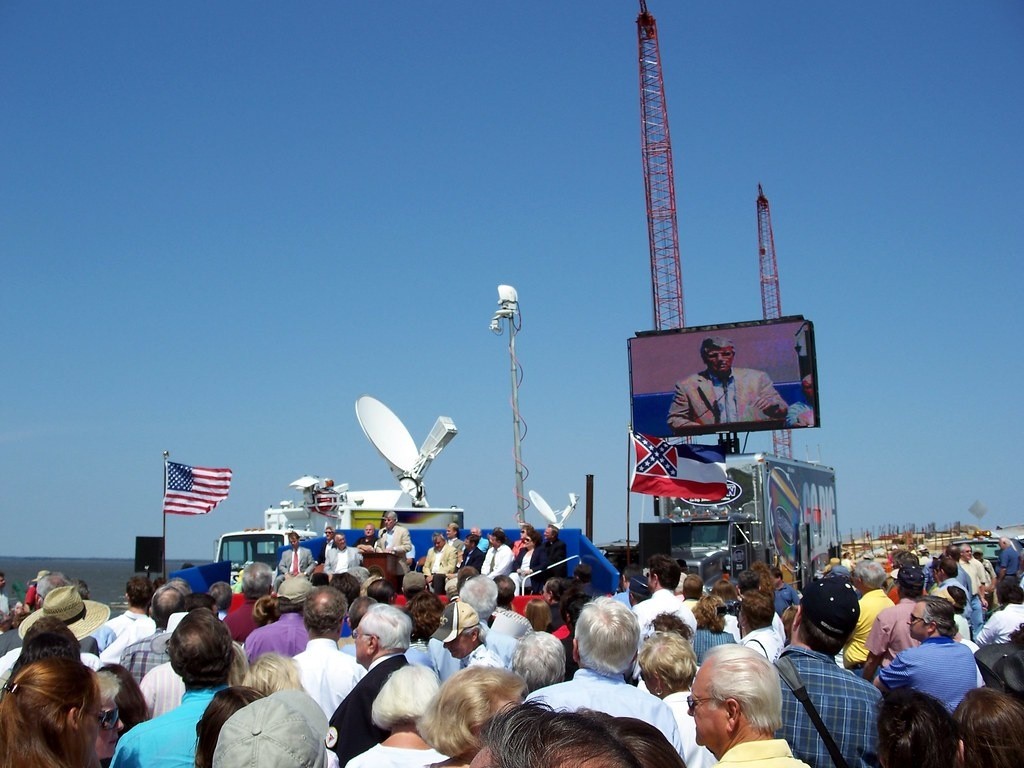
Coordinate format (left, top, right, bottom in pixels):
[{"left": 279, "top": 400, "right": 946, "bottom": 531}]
[
  {"left": 489, "top": 550, "right": 496, "bottom": 572},
  {"left": 722, "top": 377, "right": 731, "bottom": 422},
  {"left": 294, "top": 549, "right": 298, "bottom": 577}
]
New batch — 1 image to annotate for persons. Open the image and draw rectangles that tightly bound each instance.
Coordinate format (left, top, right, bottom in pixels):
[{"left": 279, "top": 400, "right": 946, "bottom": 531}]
[
  {"left": 1, "top": 511, "right": 1024, "bottom": 768},
  {"left": 667, "top": 336, "right": 788, "bottom": 426},
  {"left": 786, "top": 375, "right": 814, "bottom": 426}
]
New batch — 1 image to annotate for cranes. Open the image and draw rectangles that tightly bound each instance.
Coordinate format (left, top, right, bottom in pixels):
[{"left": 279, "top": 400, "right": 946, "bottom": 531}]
[
  {"left": 635, "top": 0, "right": 693, "bottom": 447},
  {"left": 756, "top": 183, "right": 794, "bottom": 461}
]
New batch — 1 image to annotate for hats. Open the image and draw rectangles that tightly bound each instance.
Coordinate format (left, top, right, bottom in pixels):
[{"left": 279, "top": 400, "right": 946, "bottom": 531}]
[
  {"left": 931, "top": 587, "right": 964, "bottom": 609},
  {"left": 973, "top": 548, "right": 983, "bottom": 555},
  {"left": 31, "top": 570, "right": 50, "bottom": 582},
  {"left": 929, "top": 559, "right": 941, "bottom": 569},
  {"left": 429, "top": 601, "right": 478, "bottom": 643},
  {"left": 402, "top": 571, "right": 426, "bottom": 590},
  {"left": 802, "top": 580, "right": 860, "bottom": 639},
  {"left": 676, "top": 559, "right": 686, "bottom": 566},
  {"left": 974, "top": 643, "right": 1024, "bottom": 700},
  {"left": 277, "top": 577, "right": 315, "bottom": 604},
  {"left": 212, "top": 689, "right": 329, "bottom": 768},
  {"left": 890, "top": 565, "right": 924, "bottom": 590},
  {"left": 17, "top": 586, "right": 110, "bottom": 641}
]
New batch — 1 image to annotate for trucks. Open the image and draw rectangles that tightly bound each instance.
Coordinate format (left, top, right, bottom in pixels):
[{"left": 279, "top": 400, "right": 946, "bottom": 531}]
[
  {"left": 213, "top": 490, "right": 465, "bottom": 591},
  {"left": 637, "top": 452, "right": 842, "bottom": 597}
]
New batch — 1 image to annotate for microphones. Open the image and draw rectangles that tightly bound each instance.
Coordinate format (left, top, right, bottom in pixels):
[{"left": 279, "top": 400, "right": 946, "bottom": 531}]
[{"left": 381, "top": 530, "right": 386, "bottom": 537}]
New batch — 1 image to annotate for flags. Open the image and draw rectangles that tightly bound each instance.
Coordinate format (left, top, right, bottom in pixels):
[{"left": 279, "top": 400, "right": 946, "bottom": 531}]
[
  {"left": 163, "top": 460, "right": 233, "bottom": 516},
  {"left": 630, "top": 431, "right": 728, "bottom": 499}
]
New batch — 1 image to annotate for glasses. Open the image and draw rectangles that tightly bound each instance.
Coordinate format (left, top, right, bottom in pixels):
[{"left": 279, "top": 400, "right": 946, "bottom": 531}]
[
  {"left": 98, "top": 708, "right": 120, "bottom": 729},
  {"left": 910, "top": 614, "right": 923, "bottom": 621},
  {"left": 646, "top": 573, "right": 660, "bottom": 578},
  {"left": 352, "top": 628, "right": 381, "bottom": 640},
  {"left": 687, "top": 696, "right": 739, "bottom": 710},
  {"left": 325, "top": 532, "right": 333, "bottom": 534},
  {"left": 524, "top": 539, "right": 531, "bottom": 541},
  {"left": 521, "top": 531, "right": 527, "bottom": 534}
]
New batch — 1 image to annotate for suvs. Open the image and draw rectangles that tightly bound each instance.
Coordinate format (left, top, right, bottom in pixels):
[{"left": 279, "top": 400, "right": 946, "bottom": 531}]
[{"left": 947, "top": 537, "right": 1024, "bottom": 576}]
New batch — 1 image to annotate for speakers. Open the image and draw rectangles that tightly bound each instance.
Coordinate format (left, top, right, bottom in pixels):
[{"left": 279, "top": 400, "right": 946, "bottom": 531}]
[{"left": 134, "top": 536, "right": 164, "bottom": 573}]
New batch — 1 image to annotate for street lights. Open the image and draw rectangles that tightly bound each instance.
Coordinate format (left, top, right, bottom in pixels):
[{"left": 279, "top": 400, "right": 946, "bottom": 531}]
[{"left": 489, "top": 285, "right": 526, "bottom": 526}]
